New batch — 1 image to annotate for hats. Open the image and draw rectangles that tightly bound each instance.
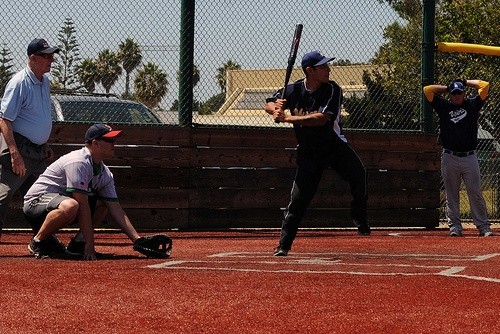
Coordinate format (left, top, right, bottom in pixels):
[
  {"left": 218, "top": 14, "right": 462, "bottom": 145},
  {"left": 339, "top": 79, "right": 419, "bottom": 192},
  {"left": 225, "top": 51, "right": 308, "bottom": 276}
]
[
  {"left": 27, "top": 38, "right": 59, "bottom": 56},
  {"left": 85, "top": 124, "right": 122, "bottom": 143},
  {"left": 448, "top": 81, "right": 464, "bottom": 92},
  {"left": 302, "top": 51, "right": 335, "bottom": 66}
]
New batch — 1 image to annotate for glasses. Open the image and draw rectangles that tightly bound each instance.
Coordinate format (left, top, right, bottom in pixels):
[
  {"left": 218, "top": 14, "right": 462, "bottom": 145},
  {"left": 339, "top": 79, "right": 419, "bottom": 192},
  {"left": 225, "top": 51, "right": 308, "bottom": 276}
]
[
  {"left": 451, "top": 90, "right": 462, "bottom": 95},
  {"left": 314, "top": 63, "right": 331, "bottom": 71},
  {"left": 95, "top": 137, "right": 116, "bottom": 143},
  {"left": 35, "top": 53, "right": 52, "bottom": 59}
]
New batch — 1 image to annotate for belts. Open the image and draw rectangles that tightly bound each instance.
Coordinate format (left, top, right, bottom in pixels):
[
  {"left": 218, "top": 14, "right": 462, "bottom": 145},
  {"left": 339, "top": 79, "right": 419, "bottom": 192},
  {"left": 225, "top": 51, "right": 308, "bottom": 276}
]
[{"left": 444, "top": 150, "right": 474, "bottom": 157}]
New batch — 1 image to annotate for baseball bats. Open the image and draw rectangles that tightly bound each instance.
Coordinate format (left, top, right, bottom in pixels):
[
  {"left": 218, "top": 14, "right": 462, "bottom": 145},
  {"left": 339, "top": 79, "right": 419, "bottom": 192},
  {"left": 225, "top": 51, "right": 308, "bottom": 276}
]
[{"left": 274, "top": 23, "right": 303, "bottom": 123}]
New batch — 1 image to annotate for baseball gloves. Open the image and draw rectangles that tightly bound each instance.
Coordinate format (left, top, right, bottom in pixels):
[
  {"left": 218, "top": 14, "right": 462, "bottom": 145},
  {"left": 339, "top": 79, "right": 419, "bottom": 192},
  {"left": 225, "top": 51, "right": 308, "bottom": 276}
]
[{"left": 132, "top": 233, "right": 173, "bottom": 258}]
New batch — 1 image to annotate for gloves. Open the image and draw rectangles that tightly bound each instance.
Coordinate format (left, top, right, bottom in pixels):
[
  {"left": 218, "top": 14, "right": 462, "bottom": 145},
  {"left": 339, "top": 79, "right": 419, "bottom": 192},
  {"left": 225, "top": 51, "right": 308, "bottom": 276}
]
[{"left": 453, "top": 77, "right": 467, "bottom": 86}]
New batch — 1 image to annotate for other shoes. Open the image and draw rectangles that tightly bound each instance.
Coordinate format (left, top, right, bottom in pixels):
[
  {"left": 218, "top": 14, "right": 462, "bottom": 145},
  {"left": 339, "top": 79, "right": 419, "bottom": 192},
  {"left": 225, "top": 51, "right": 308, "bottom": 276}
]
[
  {"left": 450, "top": 225, "right": 462, "bottom": 237},
  {"left": 274, "top": 245, "right": 288, "bottom": 256},
  {"left": 479, "top": 225, "right": 493, "bottom": 236}
]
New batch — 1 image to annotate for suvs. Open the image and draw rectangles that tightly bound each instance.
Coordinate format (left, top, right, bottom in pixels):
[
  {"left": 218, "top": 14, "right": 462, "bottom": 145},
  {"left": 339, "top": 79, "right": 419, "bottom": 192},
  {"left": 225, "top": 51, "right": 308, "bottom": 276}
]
[{"left": 50, "top": 88, "right": 165, "bottom": 124}]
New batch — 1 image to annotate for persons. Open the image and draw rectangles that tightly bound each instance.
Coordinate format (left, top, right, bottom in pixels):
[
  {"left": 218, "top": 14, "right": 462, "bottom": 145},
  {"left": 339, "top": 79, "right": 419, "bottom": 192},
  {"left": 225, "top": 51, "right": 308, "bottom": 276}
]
[
  {"left": 265, "top": 51, "right": 372, "bottom": 255},
  {"left": 0, "top": 38, "right": 173, "bottom": 261},
  {"left": 423, "top": 79, "right": 493, "bottom": 238}
]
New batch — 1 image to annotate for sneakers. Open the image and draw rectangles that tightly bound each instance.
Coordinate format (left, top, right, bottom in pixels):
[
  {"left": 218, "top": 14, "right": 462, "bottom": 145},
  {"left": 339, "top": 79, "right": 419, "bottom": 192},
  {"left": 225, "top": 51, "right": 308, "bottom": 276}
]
[
  {"left": 66, "top": 238, "right": 102, "bottom": 257},
  {"left": 28, "top": 238, "right": 54, "bottom": 258},
  {"left": 352, "top": 218, "right": 371, "bottom": 236}
]
